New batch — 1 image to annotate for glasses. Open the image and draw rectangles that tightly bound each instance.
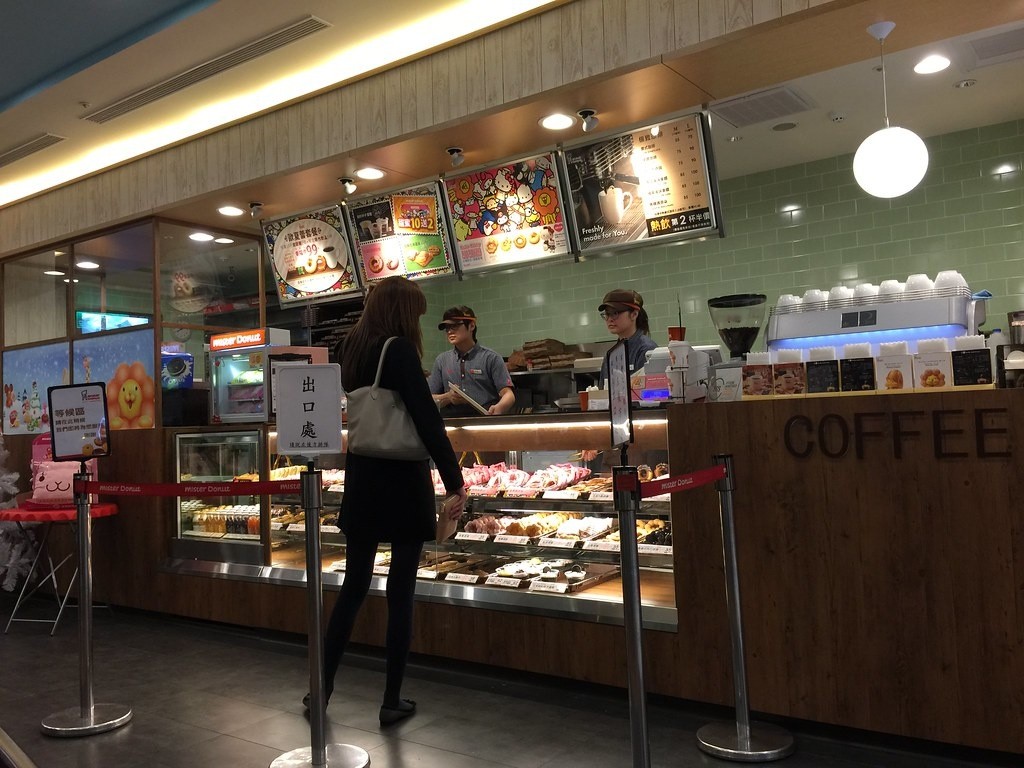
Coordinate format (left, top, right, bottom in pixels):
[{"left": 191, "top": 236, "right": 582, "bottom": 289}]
[
  {"left": 601, "top": 311, "right": 632, "bottom": 317},
  {"left": 446, "top": 323, "right": 465, "bottom": 331}
]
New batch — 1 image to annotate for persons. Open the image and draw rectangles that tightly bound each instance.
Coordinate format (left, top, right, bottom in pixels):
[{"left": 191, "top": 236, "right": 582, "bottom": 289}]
[
  {"left": 303, "top": 277, "right": 469, "bottom": 727},
  {"left": 425, "top": 305, "right": 515, "bottom": 415},
  {"left": 596, "top": 289, "right": 658, "bottom": 396}
]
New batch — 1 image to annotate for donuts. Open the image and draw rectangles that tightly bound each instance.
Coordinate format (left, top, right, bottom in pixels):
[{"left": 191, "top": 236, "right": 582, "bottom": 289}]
[
  {"left": 271, "top": 509, "right": 339, "bottom": 527},
  {"left": 464, "top": 510, "right": 673, "bottom": 547},
  {"left": 636, "top": 462, "right": 668, "bottom": 482},
  {"left": 304, "top": 256, "right": 327, "bottom": 273},
  {"left": 486, "top": 232, "right": 540, "bottom": 254},
  {"left": 369, "top": 256, "right": 383, "bottom": 273},
  {"left": 387, "top": 256, "right": 399, "bottom": 270}
]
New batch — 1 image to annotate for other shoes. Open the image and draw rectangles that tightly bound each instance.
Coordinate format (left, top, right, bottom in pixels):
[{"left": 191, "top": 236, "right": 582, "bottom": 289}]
[
  {"left": 303, "top": 693, "right": 327, "bottom": 726},
  {"left": 379, "top": 698, "right": 417, "bottom": 730}
]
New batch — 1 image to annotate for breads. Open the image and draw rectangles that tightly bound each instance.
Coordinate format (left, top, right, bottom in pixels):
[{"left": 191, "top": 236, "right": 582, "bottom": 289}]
[{"left": 250, "top": 465, "right": 308, "bottom": 479}]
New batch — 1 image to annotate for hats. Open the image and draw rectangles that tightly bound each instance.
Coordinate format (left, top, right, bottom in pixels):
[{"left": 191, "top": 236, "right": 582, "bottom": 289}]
[
  {"left": 598, "top": 289, "right": 643, "bottom": 312},
  {"left": 438, "top": 306, "right": 476, "bottom": 331}
]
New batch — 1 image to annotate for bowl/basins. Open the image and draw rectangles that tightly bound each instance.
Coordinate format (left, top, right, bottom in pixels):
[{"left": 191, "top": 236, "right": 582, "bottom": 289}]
[{"left": 773, "top": 270, "right": 971, "bottom": 316}]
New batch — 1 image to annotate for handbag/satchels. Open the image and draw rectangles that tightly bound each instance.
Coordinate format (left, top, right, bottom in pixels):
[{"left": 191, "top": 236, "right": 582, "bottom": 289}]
[{"left": 346, "top": 336, "right": 441, "bottom": 460}]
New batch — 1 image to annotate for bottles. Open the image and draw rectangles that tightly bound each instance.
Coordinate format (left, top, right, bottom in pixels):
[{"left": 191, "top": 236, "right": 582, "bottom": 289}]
[
  {"left": 527, "top": 358, "right": 533, "bottom": 371},
  {"left": 192, "top": 504, "right": 261, "bottom": 535},
  {"left": 985, "top": 328, "right": 1011, "bottom": 382}
]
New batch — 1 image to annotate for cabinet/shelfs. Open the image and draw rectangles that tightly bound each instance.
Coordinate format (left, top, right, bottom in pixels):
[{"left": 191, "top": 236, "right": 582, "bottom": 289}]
[{"left": 164, "top": 408, "right": 678, "bottom": 633}]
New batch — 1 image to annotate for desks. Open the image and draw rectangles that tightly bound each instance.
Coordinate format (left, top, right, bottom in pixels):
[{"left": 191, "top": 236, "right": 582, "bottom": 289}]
[{"left": 0, "top": 503, "right": 118, "bottom": 637}]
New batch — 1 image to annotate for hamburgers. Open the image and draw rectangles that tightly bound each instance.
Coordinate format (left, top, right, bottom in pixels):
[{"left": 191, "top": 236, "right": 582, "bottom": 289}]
[{"left": 408, "top": 245, "right": 439, "bottom": 266}]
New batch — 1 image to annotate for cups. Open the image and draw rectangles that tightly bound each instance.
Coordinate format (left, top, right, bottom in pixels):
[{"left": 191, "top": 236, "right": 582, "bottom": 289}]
[
  {"left": 667, "top": 341, "right": 690, "bottom": 367},
  {"left": 323, "top": 246, "right": 341, "bottom": 270},
  {"left": 578, "top": 391, "right": 589, "bottom": 411},
  {"left": 667, "top": 326, "right": 686, "bottom": 345},
  {"left": 664, "top": 366, "right": 688, "bottom": 405},
  {"left": 598, "top": 187, "right": 633, "bottom": 225}
]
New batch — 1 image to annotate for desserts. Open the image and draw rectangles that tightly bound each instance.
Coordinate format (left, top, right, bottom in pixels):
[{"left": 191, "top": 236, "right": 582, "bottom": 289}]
[{"left": 422, "top": 556, "right": 586, "bottom": 585}]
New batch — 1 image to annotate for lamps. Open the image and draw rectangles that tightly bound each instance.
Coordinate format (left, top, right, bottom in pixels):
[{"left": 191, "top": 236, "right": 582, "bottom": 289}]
[
  {"left": 854, "top": 22, "right": 930, "bottom": 200},
  {"left": 579, "top": 112, "right": 600, "bottom": 133},
  {"left": 251, "top": 205, "right": 263, "bottom": 218},
  {"left": 450, "top": 150, "right": 465, "bottom": 168},
  {"left": 342, "top": 179, "right": 357, "bottom": 194}
]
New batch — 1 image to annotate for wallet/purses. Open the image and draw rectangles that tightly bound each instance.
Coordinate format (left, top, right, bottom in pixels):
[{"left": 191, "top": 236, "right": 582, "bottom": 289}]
[{"left": 436, "top": 494, "right": 460, "bottom": 544}]
